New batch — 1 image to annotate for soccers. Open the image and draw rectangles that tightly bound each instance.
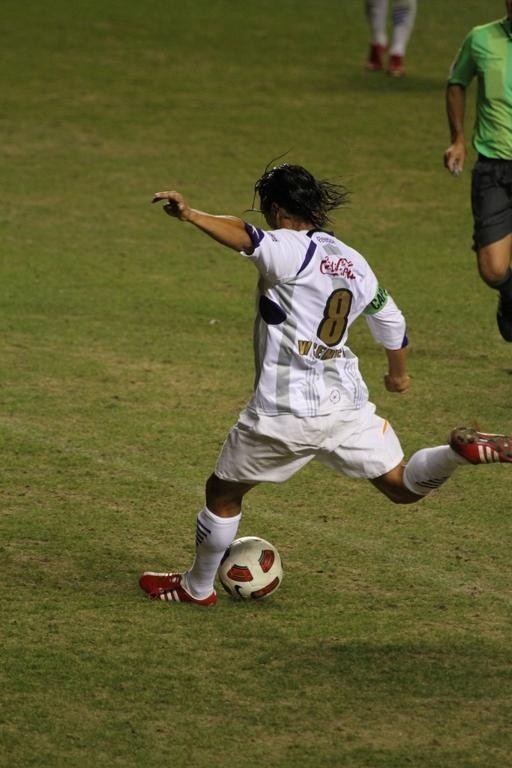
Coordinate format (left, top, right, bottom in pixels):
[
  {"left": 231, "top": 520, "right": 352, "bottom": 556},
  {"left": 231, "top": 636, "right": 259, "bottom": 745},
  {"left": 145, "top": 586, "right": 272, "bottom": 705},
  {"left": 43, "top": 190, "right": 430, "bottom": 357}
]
[{"left": 217, "top": 536, "right": 283, "bottom": 600}]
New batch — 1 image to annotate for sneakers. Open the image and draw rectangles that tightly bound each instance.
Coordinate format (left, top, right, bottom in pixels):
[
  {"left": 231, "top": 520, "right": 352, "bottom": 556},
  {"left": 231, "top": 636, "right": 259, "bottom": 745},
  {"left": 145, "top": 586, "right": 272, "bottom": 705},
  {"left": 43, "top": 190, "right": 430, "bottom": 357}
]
[
  {"left": 139, "top": 571, "right": 217, "bottom": 608},
  {"left": 448, "top": 427, "right": 512, "bottom": 465},
  {"left": 365, "top": 43, "right": 406, "bottom": 79},
  {"left": 496, "top": 283, "right": 512, "bottom": 343}
]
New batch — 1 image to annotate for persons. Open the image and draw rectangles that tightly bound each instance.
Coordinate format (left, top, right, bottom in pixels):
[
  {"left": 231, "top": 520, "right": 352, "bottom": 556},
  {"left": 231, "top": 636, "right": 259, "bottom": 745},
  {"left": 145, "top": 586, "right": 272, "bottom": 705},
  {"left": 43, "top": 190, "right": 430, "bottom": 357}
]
[
  {"left": 444, "top": 0, "right": 512, "bottom": 338},
  {"left": 364, "top": 0, "right": 418, "bottom": 77},
  {"left": 139, "top": 163, "right": 512, "bottom": 608}
]
